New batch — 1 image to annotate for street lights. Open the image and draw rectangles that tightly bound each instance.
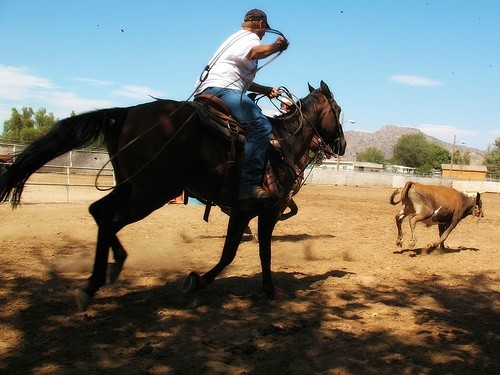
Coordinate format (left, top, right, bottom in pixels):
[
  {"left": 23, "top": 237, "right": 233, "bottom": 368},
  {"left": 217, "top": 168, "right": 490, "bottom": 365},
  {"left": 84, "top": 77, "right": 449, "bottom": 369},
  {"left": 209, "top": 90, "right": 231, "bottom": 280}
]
[
  {"left": 337, "top": 113, "right": 355, "bottom": 170},
  {"left": 450, "top": 135, "right": 465, "bottom": 178}
]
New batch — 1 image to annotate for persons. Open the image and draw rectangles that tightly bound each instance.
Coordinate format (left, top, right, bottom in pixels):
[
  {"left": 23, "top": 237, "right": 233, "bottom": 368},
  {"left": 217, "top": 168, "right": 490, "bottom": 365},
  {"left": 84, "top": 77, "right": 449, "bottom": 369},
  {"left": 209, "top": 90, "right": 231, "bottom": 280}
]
[{"left": 193, "top": 8, "right": 290, "bottom": 199}]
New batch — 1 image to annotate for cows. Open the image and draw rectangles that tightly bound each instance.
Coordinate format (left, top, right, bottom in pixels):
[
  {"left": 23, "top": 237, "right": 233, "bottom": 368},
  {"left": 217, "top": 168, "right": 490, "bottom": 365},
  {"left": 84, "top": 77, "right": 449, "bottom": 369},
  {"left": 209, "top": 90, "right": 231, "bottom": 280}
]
[{"left": 390, "top": 181, "right": 485, "bottom": 254}]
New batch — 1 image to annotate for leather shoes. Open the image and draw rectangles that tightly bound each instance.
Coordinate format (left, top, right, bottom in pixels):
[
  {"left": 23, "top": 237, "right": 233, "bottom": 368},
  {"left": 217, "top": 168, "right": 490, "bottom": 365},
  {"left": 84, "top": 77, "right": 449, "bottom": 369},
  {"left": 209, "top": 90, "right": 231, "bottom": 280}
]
[{"left": 240, "top": 186, "right": 270, "bottom": 199}]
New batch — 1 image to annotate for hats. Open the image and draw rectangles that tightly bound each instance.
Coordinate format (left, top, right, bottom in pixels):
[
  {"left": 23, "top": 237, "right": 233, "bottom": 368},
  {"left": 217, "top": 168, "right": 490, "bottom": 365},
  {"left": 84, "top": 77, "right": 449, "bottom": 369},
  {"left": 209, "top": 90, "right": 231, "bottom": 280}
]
[{"left": 245, "top": 8, "right": 271, "bottom": 29}]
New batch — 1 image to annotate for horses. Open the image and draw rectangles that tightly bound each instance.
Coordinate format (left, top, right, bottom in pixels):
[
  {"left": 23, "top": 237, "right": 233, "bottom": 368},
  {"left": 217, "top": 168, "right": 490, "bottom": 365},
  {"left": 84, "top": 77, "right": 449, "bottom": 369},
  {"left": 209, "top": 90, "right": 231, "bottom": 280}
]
[
  {"left": 1, "top": 79, "right": 347, "bottom": 311},
  {"left": 244, "top": 133, "right": 332, "bottom": 235}
]
